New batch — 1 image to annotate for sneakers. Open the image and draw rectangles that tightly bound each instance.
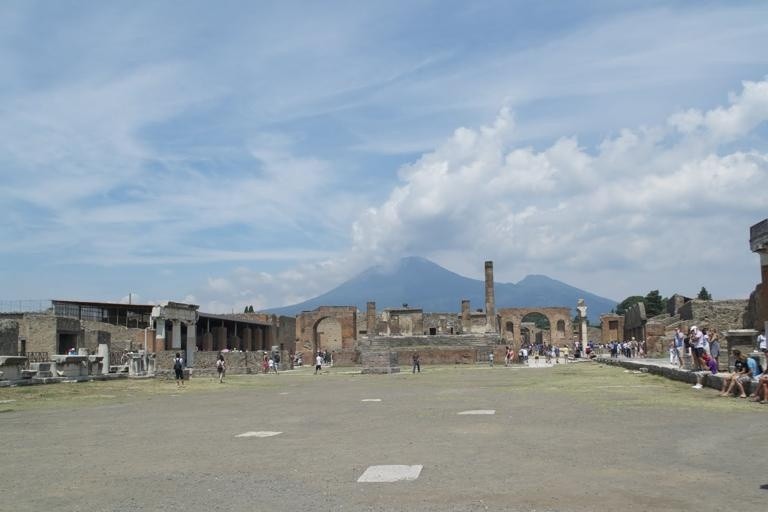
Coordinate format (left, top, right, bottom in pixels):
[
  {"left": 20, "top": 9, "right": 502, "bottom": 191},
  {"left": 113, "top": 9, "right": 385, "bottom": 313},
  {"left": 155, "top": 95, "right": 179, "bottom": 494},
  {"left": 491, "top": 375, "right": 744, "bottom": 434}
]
[
  {"left": 760, "top": 400, "right": 768, "bottom": 404},
  {"left": 692, "top": 383, "right": 698, "bottom": 388},
  {"left": 696, "top": 385, "right": 704, "bottom": 389},
  {"left": 740, "top": 394, "right": 746, "bottom": 398}
]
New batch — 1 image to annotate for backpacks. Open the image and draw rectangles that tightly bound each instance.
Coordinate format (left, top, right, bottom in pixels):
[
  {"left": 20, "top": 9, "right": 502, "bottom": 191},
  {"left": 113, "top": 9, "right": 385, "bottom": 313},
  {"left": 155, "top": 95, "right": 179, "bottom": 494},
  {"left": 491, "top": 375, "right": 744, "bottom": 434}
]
[
  {"left": 216, "top": 362, "right": 223, "bottom": 373},
  {"left": 174, "top": 358, "right": 182, "bottom": 370}
]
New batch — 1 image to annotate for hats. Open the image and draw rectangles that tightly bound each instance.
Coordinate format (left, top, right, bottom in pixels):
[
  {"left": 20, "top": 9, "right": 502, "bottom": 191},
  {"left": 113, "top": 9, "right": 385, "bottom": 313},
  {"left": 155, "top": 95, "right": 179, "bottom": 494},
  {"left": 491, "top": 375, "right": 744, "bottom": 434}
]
[
  {"left": 703, "top": 354, "right": 712, "bottom": 362},
  {"left": 690, "top": 326, "right": 697, "bottom": 332}
]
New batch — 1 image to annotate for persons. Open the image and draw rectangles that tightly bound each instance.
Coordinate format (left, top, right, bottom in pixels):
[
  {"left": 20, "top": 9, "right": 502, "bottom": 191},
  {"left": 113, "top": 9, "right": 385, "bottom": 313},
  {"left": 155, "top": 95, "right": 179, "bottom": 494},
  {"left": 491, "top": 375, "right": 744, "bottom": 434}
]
[
  {"left": 486, "top": 323, "right": 767, "bottom": 405},
  {"left": 410, "top": 349, "right": 421, "bottom": 374},
  {"left": 67, "top": 347, "right": 78, "bottom": 355},
  {"left": 172, "top": 353, "right": 187, "bottom": 388},
  {"left": 216, "top": 343, "right": 335, "bottom": 383}
]
